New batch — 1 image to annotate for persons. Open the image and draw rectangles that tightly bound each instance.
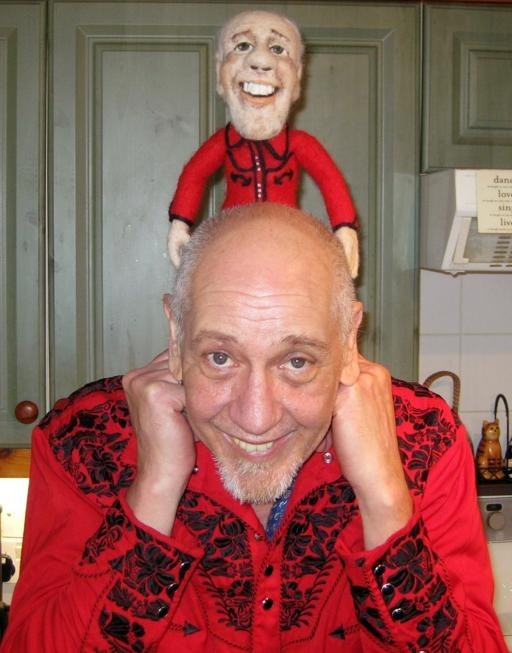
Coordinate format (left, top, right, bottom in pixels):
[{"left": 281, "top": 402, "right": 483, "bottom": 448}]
[
  {"left": 165, "top": 9, "right": 360, "bottom": 281},
  {"left": 1, "top": 201, "right": 511, "bottom": 652}
]
